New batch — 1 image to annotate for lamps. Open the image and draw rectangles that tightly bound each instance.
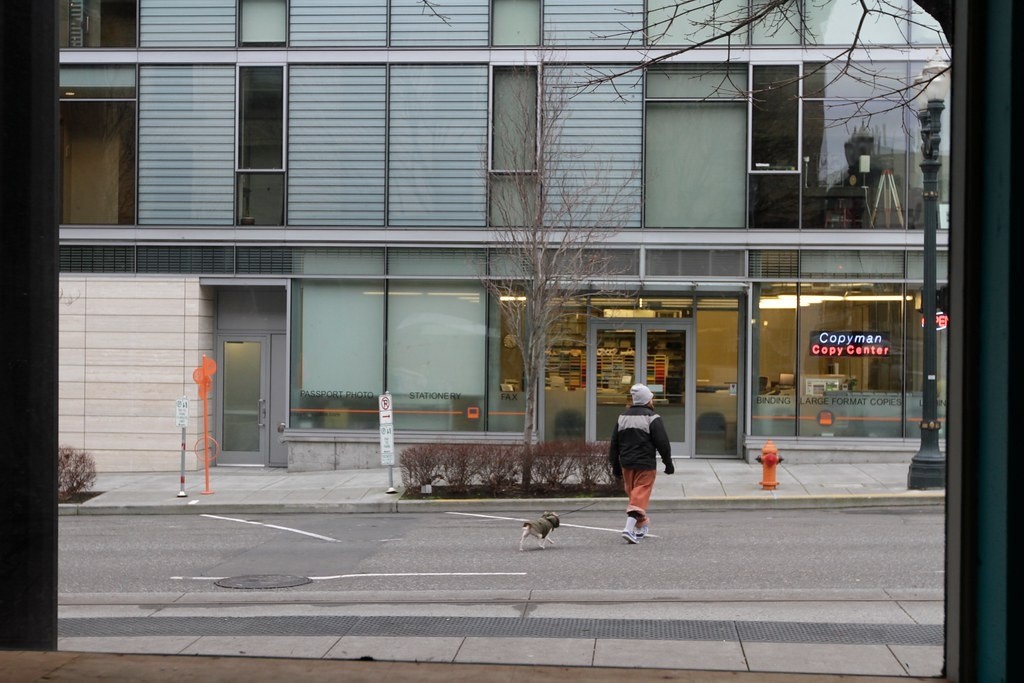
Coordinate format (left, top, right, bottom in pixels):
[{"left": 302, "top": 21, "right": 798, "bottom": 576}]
[{"left": 858, "top": 154, "right": 876, "bottom": 229}]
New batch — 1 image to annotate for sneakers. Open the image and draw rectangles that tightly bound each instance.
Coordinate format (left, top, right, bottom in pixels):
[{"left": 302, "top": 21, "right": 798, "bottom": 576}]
[
  {"left": 636, "top": 526, "right": 648, "bottom": 538},
  {"left": 621, "top": 530, "right": 639, "bottom": 543}
]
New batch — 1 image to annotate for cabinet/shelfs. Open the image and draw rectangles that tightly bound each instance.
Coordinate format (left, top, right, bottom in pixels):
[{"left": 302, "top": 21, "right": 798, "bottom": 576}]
[{"left": 543, "top": 333, "right": 685, "bottom": 406}]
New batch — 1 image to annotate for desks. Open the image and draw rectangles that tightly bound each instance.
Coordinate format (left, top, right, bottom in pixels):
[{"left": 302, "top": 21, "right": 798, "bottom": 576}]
[{"left": 802, "top": 187, "right": 870, "bottom": 229}]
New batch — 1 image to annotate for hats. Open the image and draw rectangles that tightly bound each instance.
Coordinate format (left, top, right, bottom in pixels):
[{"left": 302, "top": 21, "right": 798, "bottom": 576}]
[{"left": 630, "top": 383, "right": 654, "bottom": 406}]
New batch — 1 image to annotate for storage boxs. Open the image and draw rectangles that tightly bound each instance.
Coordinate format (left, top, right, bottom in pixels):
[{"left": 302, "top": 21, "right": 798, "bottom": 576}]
[{"left": 545, "top": 334, "right": 630, "bottom": 348}]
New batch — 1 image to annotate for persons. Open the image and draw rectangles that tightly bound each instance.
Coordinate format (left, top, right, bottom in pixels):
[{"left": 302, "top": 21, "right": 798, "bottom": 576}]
[{"left": 608, "top": 383, "right": 674, "bottom": 544}]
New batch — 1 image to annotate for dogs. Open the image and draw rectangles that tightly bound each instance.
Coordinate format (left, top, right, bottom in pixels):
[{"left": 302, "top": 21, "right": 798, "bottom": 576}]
[{"left": 519, "top": 511, "right": 559, "bottom": 552}]
